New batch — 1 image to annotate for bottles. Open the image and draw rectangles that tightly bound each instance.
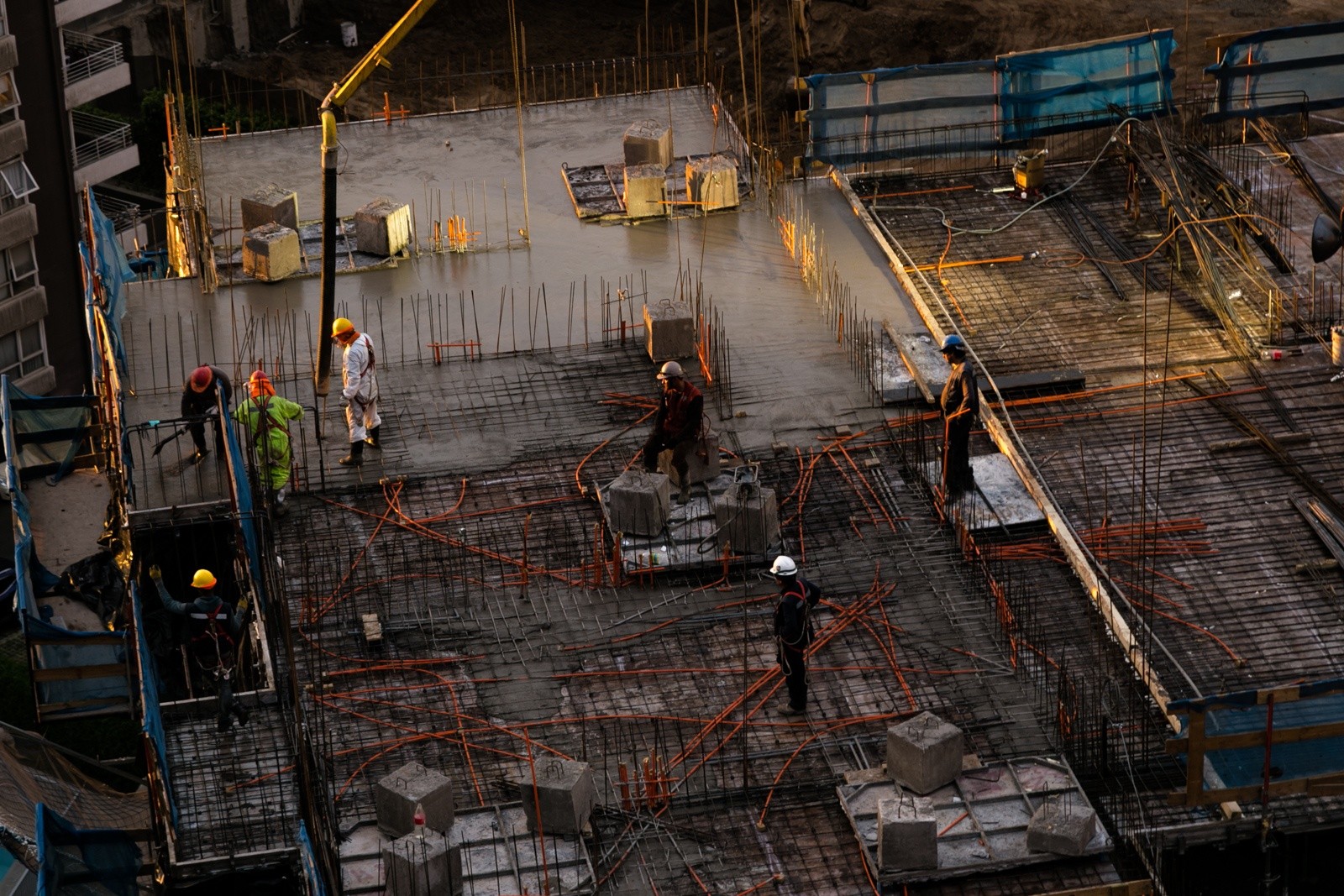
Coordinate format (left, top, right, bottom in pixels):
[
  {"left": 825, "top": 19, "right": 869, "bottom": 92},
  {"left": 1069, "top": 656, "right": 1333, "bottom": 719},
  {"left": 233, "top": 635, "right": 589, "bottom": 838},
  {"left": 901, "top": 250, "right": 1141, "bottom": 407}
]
[
  {"left": 1261, "top": 350, "right": 1292, "bottom": 360},
  {"left": 413, "top": 803, "right": 427, "bottom": 839}
]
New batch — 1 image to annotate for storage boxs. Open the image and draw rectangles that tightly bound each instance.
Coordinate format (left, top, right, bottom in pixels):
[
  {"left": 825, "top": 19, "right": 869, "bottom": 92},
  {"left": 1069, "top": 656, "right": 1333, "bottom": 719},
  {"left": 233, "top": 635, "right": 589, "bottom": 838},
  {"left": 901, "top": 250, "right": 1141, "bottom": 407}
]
[{"left": 1015, "top": 149, "right": 1047, "bottom": 192}]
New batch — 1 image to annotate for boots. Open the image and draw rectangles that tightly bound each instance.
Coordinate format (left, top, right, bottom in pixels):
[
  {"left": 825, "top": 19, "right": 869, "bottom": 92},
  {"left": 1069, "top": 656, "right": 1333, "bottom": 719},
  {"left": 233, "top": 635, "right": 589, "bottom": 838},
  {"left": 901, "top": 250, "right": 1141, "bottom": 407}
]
[
  {"left": 339, "top": 438, "right": 364, "bottom": 466},
  {"left": 365, "top": 423, "right": 381, "bottom": 447}
]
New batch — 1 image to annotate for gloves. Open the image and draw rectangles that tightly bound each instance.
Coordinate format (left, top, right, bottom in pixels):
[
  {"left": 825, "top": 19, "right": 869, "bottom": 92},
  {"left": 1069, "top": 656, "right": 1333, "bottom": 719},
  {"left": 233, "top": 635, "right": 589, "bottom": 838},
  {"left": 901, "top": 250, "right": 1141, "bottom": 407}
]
[
  {"left": 237, "top": 595, "right": 248, "bottom": 611},
  {"left": 148, "top": 565, "right": 161, "bottom": 581}
]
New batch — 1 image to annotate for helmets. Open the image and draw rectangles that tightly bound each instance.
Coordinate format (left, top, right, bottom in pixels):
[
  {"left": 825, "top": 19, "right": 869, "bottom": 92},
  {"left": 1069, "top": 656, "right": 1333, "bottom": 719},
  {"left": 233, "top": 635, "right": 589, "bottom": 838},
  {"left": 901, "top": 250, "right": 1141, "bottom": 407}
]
[
  {"left": 770, "top": 555, "right": 797, "bottom": 576},
  {"left": 190, "top": 366, "right": 213, "bottom": 393},
  {"left": 938, "top": 335, "right": 962, "bottom": 352},
  {"left": 330, "top": 317, "right": 354, "bottom": 338},
  {"left": 190, "top": 569, "right": 217, "bottom": 588},
  {"left": 656, "top": 362, "right": 683, "bottom": 380},
  {"left": 242, "top": 371, "right": 270, "bottom": 385}
]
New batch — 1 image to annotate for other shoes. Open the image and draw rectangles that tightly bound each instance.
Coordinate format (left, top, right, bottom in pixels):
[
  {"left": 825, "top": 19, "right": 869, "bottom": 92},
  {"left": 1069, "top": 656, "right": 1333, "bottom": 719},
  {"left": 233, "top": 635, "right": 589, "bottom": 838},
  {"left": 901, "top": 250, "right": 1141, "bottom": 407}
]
[
  {"left": 218, "top": 717, "right": 235, "bottom": 733},
  {"left": 944, "top": 490, "right": 961, "bottom": 503},
  {"left": 194, "top": 448, "right": 206, "bottom": 462},
  {"left": 235, "top": 704, "right": 251, "bottom": 726},
  {"left": 677, "top": 489, "right": 690, "bottom": 503},
  {"left": 778, "top": 701, "right": 805, "bottom": 715}
]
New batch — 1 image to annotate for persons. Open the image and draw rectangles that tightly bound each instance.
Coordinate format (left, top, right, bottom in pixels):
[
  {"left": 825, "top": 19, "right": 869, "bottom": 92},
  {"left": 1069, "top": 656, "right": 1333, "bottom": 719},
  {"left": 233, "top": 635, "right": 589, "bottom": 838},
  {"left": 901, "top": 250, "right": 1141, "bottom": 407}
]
[
  {"left": 938, "top": 334, "right": 979, "bottom": 502},
  {"left": 181, "top": 364, "right": 232, "bottom": 462},
  {"left": 233, "top": 370, "right": 304, "bottom": 508},
  {"left": 768, "top": 555, "right": 821, "bottom": 715},
  {"left": 150, "top": 565, "right": 252, "bottom": 730},
  {"left": 641, "top": 361, "right": 704, "bottom": 505},
  {"left": 330, "top": 318, "right": 382, "bottom": 465}
]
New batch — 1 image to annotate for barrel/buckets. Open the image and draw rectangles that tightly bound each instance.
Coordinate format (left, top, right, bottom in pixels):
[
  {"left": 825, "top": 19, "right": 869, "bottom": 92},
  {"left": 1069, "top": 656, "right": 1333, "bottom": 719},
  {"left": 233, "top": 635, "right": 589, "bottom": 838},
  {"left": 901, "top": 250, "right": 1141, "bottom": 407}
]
[{"left": 1331, "top": 326, "right": 1344, "bottom": 367}]
